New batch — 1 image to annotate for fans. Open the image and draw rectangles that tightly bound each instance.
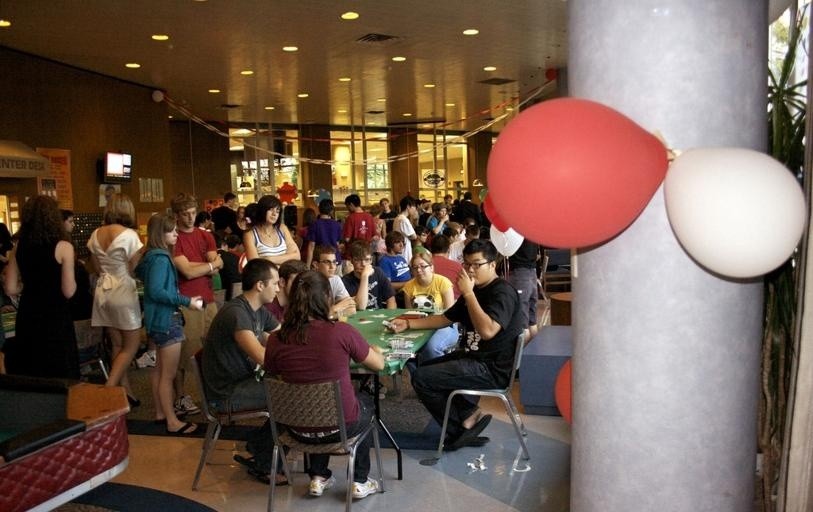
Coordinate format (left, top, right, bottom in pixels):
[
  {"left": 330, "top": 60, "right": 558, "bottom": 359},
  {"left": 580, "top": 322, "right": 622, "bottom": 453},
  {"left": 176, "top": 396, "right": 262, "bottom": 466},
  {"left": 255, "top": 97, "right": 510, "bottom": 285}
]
[{"left": 424, "top": 169, "right": 447, "bottom": 187}]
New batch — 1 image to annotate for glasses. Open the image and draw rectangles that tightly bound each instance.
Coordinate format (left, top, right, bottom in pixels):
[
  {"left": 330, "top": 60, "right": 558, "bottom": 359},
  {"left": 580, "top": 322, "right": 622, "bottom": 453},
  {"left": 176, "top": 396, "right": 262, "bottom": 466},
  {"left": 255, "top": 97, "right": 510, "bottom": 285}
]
[
  {"left": 411, "top": 265, "right": 429, "bottom": 271},
  {"left": 462, "top": 262, "right": 489, "bottom": 269}
]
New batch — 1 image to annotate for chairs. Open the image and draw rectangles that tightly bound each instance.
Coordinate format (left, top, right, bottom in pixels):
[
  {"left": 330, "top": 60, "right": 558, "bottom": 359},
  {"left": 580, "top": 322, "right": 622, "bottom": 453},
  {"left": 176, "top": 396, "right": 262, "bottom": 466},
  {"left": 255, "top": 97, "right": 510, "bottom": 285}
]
[
  {"left": 72, "top": 318, "right": 111, "bottom": 386},
  {"left": 435, "top": 329, "right": 532, "bottom": 469},
  {"left": 527, "top": 243, "right": 571, "bottom": 328},
  {"left": 179, "top": 350, "right": 290, "bottom": 492},
  {"left": 259, "top": 374, "right": 386, "bottom": 512}
]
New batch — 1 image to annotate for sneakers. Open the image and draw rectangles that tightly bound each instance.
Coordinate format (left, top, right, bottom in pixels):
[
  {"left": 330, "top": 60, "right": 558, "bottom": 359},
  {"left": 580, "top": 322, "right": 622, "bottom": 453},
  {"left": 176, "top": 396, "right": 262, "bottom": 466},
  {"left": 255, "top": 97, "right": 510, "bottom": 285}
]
[
  {"left": 234, "top": 379, "right": 492, "bottom": 499},
  {"left": 124, "top": 350, "right": 202, "bottom": 437}
]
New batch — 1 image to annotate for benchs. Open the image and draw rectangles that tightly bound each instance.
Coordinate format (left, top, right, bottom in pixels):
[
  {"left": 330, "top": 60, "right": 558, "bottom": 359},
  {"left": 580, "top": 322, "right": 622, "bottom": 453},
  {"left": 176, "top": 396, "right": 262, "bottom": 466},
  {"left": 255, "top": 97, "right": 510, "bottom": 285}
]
[{"left": 517, "top": 319, "right": 571, "bottom": 418}]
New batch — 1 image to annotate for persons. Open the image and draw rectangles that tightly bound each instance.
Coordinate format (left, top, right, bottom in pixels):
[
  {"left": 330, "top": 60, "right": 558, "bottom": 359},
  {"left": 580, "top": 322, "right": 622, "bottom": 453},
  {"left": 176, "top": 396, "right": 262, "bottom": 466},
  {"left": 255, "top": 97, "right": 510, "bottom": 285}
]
[
  {"left": 385, "top": 236, "right": 524, "bottom": 454},
  {"left": 172, "top": 192, "right": 543, "bottom": 411},
  {"left": 132, "top": 207, "right": 208, "bottom": 438},
  {"left": 1, "top": 220, "right": 15, "bottom": 357},
  {"left": 83, "top": 190, "right": 145, "bottom": 410},
  {"left": 198, "top": 255, "right": 294, "bottom": 487},
  {"left": 263, "top": 268, "right": 386, "bottom": 504},
  {"left": 57, "top": 206, "right": 79, "bottom": 238},
  {"left": 3, "top": 192, "right": 81, "bottom": 386}
]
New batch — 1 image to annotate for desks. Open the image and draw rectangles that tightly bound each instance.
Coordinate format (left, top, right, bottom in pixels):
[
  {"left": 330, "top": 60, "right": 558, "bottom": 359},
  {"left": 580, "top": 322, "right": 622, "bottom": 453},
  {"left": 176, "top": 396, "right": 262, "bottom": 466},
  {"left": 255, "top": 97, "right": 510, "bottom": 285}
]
[
  {"left": 331, "top": 303, "right": 444, "bottom": 481},
  {"left": 0, "top": 302, "right": 18, "bottom": 345},
  {"left": 0, "top": 371, "right": 132, "bottom": 512}
]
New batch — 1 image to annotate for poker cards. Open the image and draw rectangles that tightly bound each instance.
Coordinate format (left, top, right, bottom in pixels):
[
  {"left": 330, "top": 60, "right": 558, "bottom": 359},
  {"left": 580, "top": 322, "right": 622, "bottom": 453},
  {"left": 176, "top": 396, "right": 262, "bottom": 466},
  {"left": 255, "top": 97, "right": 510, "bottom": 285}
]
[{"left": 381, "top": 320, "right": 398, "bottom": 331}]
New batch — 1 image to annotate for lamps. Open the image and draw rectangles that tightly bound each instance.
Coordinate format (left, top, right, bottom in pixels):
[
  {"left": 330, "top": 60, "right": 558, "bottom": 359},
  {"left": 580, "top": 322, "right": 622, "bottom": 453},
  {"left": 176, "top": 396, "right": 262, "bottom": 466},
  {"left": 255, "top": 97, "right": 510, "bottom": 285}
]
[
  {"left": 303, "top": 121, "right": 321, "bottom": 199},
  {"left": 469, "top": 130, "right": 484, "bottom": 188},
  {"left": 238, "top": 165, "right": 253, "bottom": 190}
]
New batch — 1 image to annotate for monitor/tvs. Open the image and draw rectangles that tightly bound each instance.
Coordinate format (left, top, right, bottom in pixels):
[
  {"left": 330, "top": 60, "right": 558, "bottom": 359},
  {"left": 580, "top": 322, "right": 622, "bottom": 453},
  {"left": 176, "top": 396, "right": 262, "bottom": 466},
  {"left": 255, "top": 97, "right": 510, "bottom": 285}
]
[{"left": 104, "top": 152, "right": 132, "bottom": 183}]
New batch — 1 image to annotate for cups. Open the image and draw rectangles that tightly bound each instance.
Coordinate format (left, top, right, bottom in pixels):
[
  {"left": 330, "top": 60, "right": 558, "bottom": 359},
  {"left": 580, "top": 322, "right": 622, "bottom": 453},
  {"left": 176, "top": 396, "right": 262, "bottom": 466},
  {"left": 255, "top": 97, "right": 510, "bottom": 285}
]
[
  {"left": 195, "top": 300, "right": 204, "bottom": 309},
  {"left": 336, "top": 308, "right": 348, "bottom": 324}
]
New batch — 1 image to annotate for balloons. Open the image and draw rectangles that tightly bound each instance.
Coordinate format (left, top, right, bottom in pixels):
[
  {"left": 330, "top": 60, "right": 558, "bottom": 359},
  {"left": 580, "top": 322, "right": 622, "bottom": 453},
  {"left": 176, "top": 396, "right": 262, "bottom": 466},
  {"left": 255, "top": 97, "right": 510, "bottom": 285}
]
[
  {"left": 479, "top": 93, "right": 669, "bottom": 255},
  {"left": 661, "top": 143, "right": 809, "bottom": 283}
]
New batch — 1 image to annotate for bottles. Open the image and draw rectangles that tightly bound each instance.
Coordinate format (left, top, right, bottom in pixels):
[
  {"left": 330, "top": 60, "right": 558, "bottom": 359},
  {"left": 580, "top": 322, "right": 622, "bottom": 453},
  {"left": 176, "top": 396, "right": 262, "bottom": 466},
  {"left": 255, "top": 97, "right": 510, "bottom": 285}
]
[{"left": 70, "top": 211, "right": 104, "bottom": 258}]
